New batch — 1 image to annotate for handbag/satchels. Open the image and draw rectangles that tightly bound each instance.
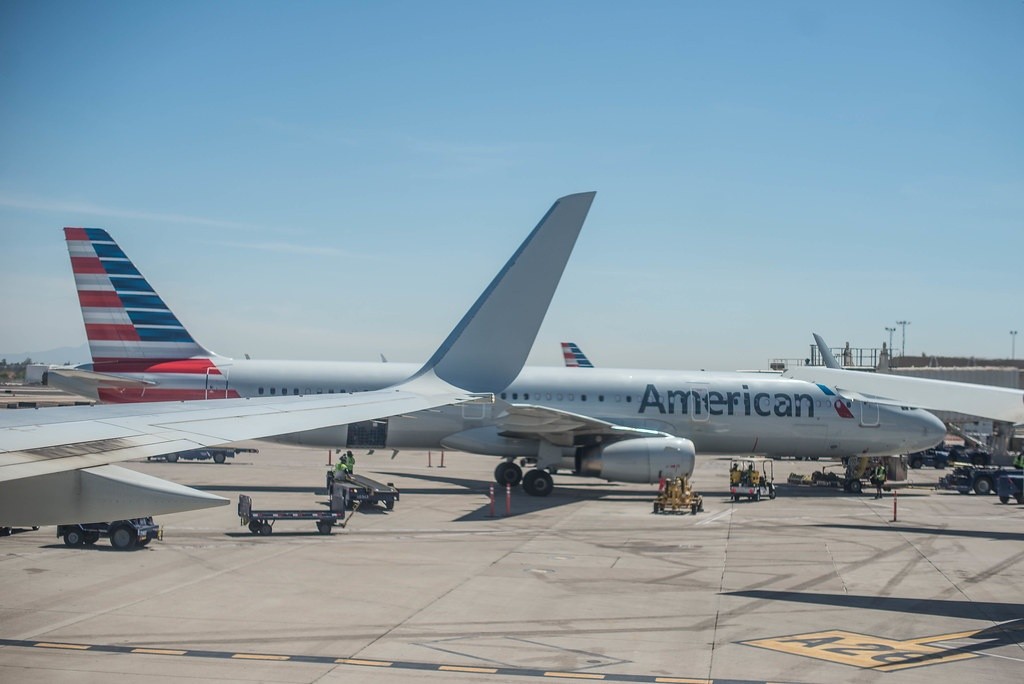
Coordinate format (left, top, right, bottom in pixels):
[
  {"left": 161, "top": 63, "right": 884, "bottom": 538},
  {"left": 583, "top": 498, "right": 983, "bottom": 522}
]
[
  {"left": 883, "top": 485, "right": 891, "bottom": 491},
  {"left": 870, "top": 475, "right": 877, "bottom": 484}
]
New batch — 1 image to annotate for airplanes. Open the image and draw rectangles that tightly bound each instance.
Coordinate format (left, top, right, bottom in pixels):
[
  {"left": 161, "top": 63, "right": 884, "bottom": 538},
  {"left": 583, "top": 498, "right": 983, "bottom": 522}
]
[
  {"left": 0, "top": 189, "right": 597, "bottom": 530},
  {"left": 46, "top": 226, "right": 949, "bottom": 497}
]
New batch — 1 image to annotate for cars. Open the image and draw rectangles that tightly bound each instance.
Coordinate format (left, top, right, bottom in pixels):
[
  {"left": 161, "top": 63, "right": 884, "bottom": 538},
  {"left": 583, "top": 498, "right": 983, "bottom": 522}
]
[{"left": 56, "top": 516, "right": 164, "bottom": 550}]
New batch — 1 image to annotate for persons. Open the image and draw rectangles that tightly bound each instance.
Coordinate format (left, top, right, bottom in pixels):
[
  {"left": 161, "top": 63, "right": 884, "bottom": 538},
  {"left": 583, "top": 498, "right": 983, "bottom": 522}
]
[
  {"left": 337, "top": 451, "right": 356, "bottom": 482},
  {"left": 1014, "top": 452, "right": 1024, "bottom": 471},
  {"left": 730, "top": 464, "right": 753, "bottom": 486},
  {"left": 874, "top": 462, "right": 888, "bottom": 499}
]
